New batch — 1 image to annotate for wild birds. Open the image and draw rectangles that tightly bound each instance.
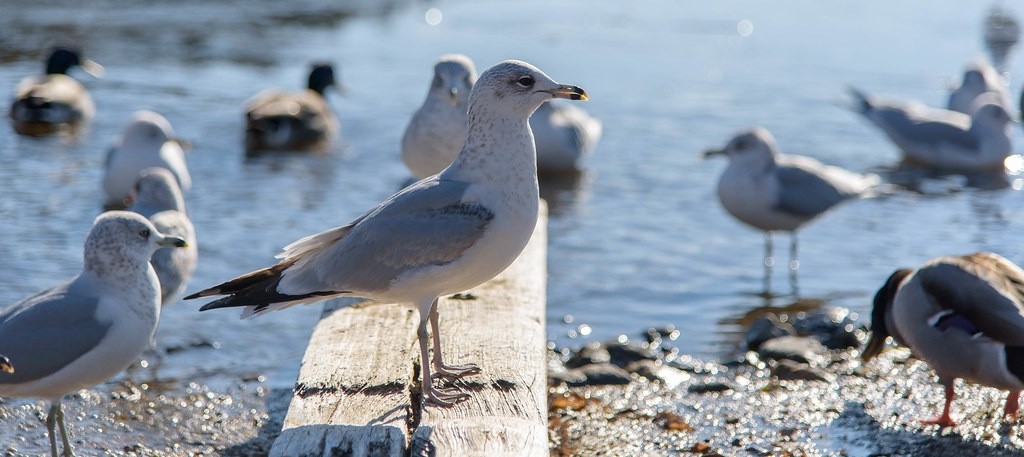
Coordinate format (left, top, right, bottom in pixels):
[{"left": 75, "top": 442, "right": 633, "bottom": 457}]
[
  {"left": 5, "top": 38, "right": 105, "bottom": 138},
  {"left": 0, "top": 104, "right": 200, "bottom": 457},
  {"left": 844, "top": 12, "right": 1023, "bottom": 187},
  {"left": 704, "top": 126, "right": 880, "bottom": 256},
  {"left": 181, "top": 57, "right": 603, "bottom": 409},
  {"left": 861, "top": 251, "right": 1023, "bottom": 425}
]
